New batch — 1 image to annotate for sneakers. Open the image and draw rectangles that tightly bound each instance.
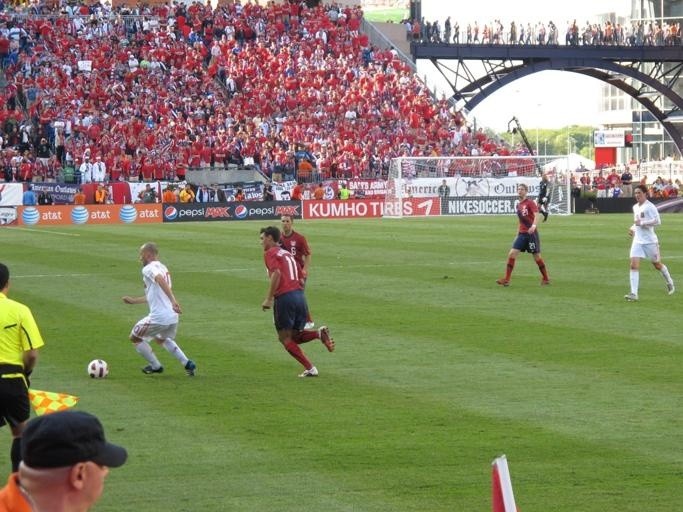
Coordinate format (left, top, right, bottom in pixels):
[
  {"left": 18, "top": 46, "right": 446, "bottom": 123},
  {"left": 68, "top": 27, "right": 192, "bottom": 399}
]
[
  {"left": 318, "top": 325, "right": 336, "bottom": 352},
  {"left": 623, "top": 293, "right": 638, "bottom": 300},
  {"left": 143, "top": 366, "right": 164, "bottom": 374},
  {"left": 496, "top": 279, "right": 509, "bottom": 286},
  {"left": 298, "top": 366, "right": 318, "bottom": 378},
  {"left": 185, "top": 360, "right": 196, "bottom": 375},
  {"left": 304, "top": 320, "right": 314, "bottom": 329},
  {"left": 667, "top": 283, "right": 675, "bottom": 296}
]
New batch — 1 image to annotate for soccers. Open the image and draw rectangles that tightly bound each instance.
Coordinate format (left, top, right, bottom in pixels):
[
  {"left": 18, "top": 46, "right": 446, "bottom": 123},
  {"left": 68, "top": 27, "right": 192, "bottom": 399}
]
[
  {"left": 71, "top": 206, "right": 89, "bottom": 224},
  {"left": 22, "top": 208, "right": 41, "bottom": 225},
  {"left": 88, "top": 359, "right": 109, "bottom": 377},
  {"left": 120, "top": 205, "right": 138, "bottom": 222}
]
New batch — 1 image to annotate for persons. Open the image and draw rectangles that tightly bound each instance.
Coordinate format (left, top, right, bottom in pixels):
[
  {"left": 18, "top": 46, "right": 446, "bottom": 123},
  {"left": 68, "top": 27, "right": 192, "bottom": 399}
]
[
  {"left": 0, "top": 0, "right": 538, "bottom": 207},
  {"left": 259, "top": 226, "right": 335, "bottom": 378},
  {"left": 277, "top": 213, "right": 310, "bottom": 290},
  {"left": 624, "top": 185, "right": 675, "bottom": 300},
  {"left": 496, "top": 183, "right": 550, "bottom": 286},
  {"left": 0, "top": 263, "right": 45, "bottom": 437},
  {"left": 399, "top": 14, "right": 682, "bottom": 47},
  {"left": 121, "top": 243, "right": 196, "bottom": 377},
  {"left": 545, "top": 153, "right": 682, "bottom": 199},
  {"left": 0, "top": 411, "right": 128, "bottom": 512}
]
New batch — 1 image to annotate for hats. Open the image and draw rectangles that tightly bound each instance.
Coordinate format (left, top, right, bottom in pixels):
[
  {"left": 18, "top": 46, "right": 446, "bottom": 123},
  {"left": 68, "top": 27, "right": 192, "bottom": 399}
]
[{"left": 21, "top": 409, "right": 127, "bottom": 467}]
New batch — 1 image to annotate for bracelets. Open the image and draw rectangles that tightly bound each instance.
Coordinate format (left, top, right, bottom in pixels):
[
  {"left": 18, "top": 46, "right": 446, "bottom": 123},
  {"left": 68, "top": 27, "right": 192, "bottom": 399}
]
[{"left": 532, "top": 224, "right": 537, "bottom": 228}]
